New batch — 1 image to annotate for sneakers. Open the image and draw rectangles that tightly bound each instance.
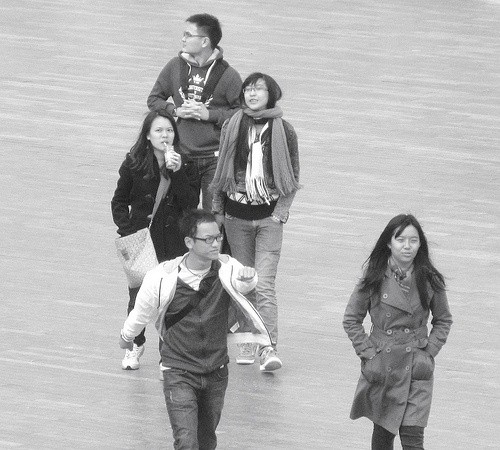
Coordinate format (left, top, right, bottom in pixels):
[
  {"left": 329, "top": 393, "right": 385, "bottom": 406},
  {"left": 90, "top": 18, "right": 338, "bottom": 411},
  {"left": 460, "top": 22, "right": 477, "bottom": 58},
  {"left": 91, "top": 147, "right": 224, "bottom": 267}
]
[
  {"left": 236, "top": 342, "right": 257, "bottom": 364},
  {"left": 259, "top": 347, "right": 281, "bottom": 371},
  {"left": 122, "top": 343, "right": 144, "bottom": 369},
  {"left": 159, "top": 369, "right": 164, "bottom": 381}
]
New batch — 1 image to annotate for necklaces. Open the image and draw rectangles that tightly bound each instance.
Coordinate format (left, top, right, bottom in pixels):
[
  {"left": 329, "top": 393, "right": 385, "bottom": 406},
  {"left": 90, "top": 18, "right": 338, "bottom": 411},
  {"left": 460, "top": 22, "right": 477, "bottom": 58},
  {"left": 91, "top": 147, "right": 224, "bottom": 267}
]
[{"left": 185, "top": 255, "right": 212, "bottom": 279}]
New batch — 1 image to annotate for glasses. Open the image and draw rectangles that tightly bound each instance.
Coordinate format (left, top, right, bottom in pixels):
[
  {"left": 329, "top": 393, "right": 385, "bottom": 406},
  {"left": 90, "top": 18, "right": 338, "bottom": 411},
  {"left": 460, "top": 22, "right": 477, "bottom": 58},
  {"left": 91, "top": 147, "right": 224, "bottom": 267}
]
[
  {"left": 183, "top": 32, "right": 209, "bottom": 39},
  {"left": 242, "top": 87, "right": 269, "bottom": 93},
  {"left": 191, "top": 234, "right": 224, "bottom": 244}
]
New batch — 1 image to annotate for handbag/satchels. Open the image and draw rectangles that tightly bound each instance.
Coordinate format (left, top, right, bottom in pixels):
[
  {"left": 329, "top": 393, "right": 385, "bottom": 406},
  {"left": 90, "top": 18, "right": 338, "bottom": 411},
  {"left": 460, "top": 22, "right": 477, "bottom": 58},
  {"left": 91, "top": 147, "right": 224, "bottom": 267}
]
[{"left": 115, "top": 227, "right": 159, "bottom": 289}]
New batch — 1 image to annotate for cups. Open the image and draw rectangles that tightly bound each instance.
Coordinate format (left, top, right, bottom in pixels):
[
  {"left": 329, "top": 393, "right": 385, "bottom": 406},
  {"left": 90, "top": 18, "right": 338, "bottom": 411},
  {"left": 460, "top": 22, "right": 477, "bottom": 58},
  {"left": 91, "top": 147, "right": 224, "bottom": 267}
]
[{"left": 165, "top": 145, "right": 176, "bottom": 170}]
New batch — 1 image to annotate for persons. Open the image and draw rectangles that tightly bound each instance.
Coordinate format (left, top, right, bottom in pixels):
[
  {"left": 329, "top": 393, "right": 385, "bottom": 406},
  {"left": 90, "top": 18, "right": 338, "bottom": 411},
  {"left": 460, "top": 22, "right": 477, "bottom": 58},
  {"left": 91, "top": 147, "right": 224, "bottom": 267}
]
[
  {"left": 110, "top": 109, "right": 201, "bottom": 380},
  {"left": 208, "top": 72, "right": 303, "bottom": 373},
  {"left": 343, "top": 214, "right": 454, "bottom": 450},
  {"left": 147, "top": 14, "right": 244, "bottom": 261},
  {"left": 117, "top": 208, "right": 258, "bottom": 450}
]
